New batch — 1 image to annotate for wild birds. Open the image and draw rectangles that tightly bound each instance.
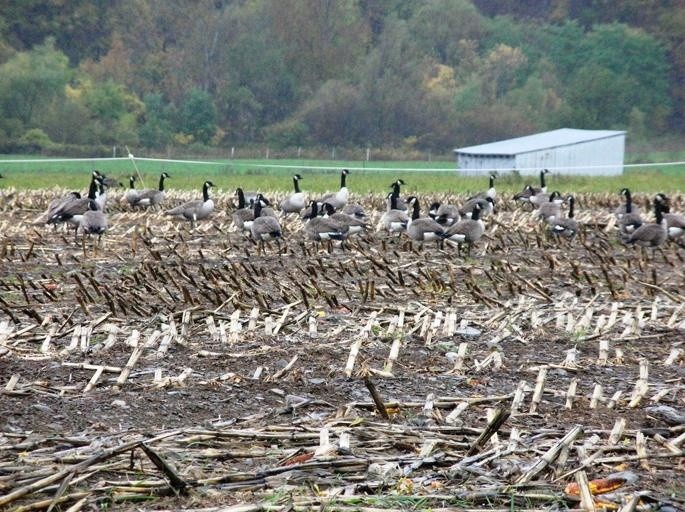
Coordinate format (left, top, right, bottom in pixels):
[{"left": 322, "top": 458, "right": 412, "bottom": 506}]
[
  {"left": 509, "top": 168, "right": 584, "bottom": 253},
  {"left": 226, "top": 171, "right": 500, "bottom": 257},
  {"left": 606, "top": 188, "right": 684, "bottom": 255},
  {"left": 32, "top": 169, "right": 110, "bottom": 254},
  {"left": 165, "top": 180, "right": 217, "bottom": 232},
  {"left": 119, "top": 172, "right": 173, "bottom": 211}
]
